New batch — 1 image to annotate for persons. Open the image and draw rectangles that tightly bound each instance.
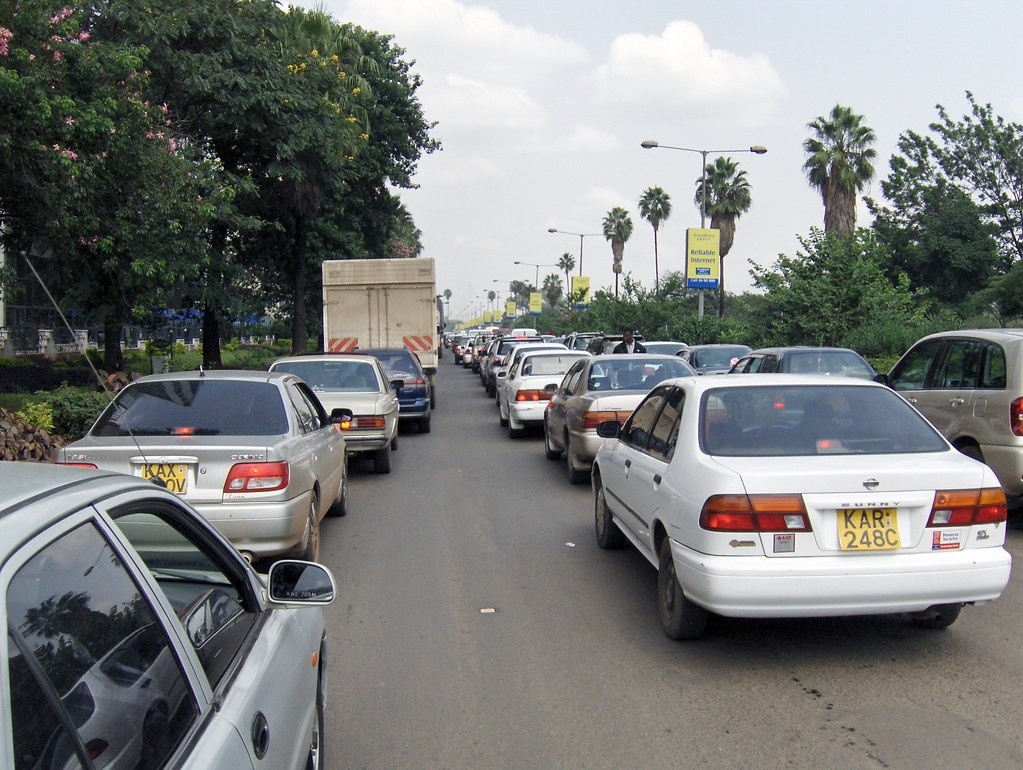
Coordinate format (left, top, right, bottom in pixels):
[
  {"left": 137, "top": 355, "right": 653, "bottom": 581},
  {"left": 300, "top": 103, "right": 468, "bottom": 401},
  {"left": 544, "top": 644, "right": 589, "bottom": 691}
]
[
  {"left": 613, "top": 328, "right": 646, "bottom": 386},
  {"left": 357, "top": 365, "right": 373, "bottom": 386},
  {"left": 560, "top": 330, "right": 567, "bottom": 337},
  {"left": 480, "top": 334, "right": 503, "bottom": 345}
]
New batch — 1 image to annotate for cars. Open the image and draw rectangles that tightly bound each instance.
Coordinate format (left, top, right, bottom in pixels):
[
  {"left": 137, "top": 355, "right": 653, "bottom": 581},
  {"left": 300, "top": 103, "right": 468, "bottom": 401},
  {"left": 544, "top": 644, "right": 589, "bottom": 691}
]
[
  {"left": 267, "top": 355, "right": 405, "bottom": 474},
  {"left": 0, "top": 460, "right": 338, "bottom": 770},
  {"left": 872, "top": 329, "right": 1023, "bottom": 510},
  {"left": 673, "top": 344, "right": 763, "bottom": 376},
  {"left": 443, "top": 327, "right": 689, "bottom": 406},
  {"left": 722, "top": 346, "right": 878, "bottom": 424},
  {"left": 543, "top": 354, "right": 727, "bottom": 484},
  {"left": 56, "top": 370, "right": 354, "bottom": 563},
  {"left": 591, "top": 374, "right": 1011, "bottom": 640}
]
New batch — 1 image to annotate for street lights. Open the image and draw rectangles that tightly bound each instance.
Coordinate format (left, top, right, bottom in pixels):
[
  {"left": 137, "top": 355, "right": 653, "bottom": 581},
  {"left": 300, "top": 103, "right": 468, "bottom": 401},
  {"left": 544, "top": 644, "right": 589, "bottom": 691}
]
[
  {"left": 548, "top": 228, "right": 617, "bottom": 277},
  {"left": 514, "top": 261, "right": 562, "bottom": 328},
  {"left": 458, "top": 290, "right": 501, "bottom": 322},
  {"left": 641, "top": 140, "right": 767, "bottom": 321},
  {"left": 493, "top": 279, "right": 530, "bottom": 318}
]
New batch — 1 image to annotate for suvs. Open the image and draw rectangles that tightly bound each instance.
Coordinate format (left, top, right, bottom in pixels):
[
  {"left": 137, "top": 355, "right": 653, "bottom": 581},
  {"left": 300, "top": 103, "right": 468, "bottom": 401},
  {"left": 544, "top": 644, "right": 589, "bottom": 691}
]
[{"left": 350, "top": 346, "right": 438, "bottom": 434}]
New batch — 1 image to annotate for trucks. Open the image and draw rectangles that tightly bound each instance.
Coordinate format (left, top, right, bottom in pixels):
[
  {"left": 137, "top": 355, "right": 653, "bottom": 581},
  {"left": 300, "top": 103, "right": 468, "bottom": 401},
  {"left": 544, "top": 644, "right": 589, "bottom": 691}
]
[{"left": 321, "top": 257, "right": 438, "bottom": 410}]
